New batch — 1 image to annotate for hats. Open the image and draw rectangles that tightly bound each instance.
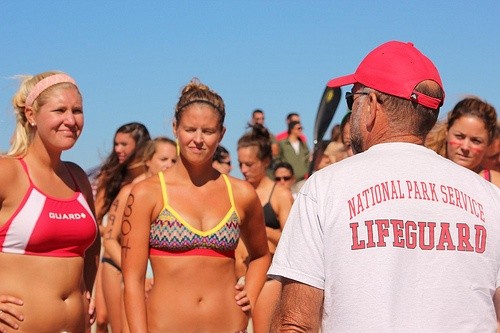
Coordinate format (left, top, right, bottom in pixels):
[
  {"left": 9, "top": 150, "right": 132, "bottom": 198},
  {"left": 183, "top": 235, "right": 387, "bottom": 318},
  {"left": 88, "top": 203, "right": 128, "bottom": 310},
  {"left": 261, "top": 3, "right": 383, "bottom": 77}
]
[{"left": 327, "top": 40, "right": 446, "bottom": 109}]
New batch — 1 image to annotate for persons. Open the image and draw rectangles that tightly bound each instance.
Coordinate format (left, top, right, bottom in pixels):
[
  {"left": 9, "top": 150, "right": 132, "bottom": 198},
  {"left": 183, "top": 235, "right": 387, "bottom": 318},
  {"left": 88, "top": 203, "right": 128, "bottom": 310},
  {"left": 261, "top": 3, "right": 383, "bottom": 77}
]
[
  {"left": 274, "top": 162, "right": 297, "bottom": 189},
  {"left": 275, "top": 114, "right": 306, "bottom": 142},
  {"left": 120, "top": 82, "right": 272, "bottom": 333},
  {"left": 265, "top": 41, "right": 500, "bottom": 333},
  {"left": 95, "top": 123, "right": 151, "bottom": 333},
  {"left": 102, "top": 138, "right": 178, "bottom": 333},
  {"left": 212, "top": 146, "right": 232, "bottom": 174},
  {"left": 316, "top": 112, "right": 354, "bottom": 169},
  {"left": 253, "top": 110, "right": 279, "bottom": 181},
  {"left": 426, "top": 97, "right": 500, "bottom": 187},
  {"left": 237, "top": 123, "right": 293, "bottom": 333},
  {"left": 279, "top": 120, "right": 310, "bottom": 180},
  {"left": 0, "top": 71, "right": 101, "bottom": 333}
]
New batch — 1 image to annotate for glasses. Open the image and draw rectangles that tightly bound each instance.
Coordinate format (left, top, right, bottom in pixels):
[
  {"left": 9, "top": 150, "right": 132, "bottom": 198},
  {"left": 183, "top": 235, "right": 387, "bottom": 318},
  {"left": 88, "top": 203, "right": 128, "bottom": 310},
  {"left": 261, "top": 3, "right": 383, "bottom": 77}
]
[
  {"left": 345, "top": 91, "right": 384, "bottom": 111},
  {"left": 273, "top": 174, "right": 292, "bottom": 181}
]
